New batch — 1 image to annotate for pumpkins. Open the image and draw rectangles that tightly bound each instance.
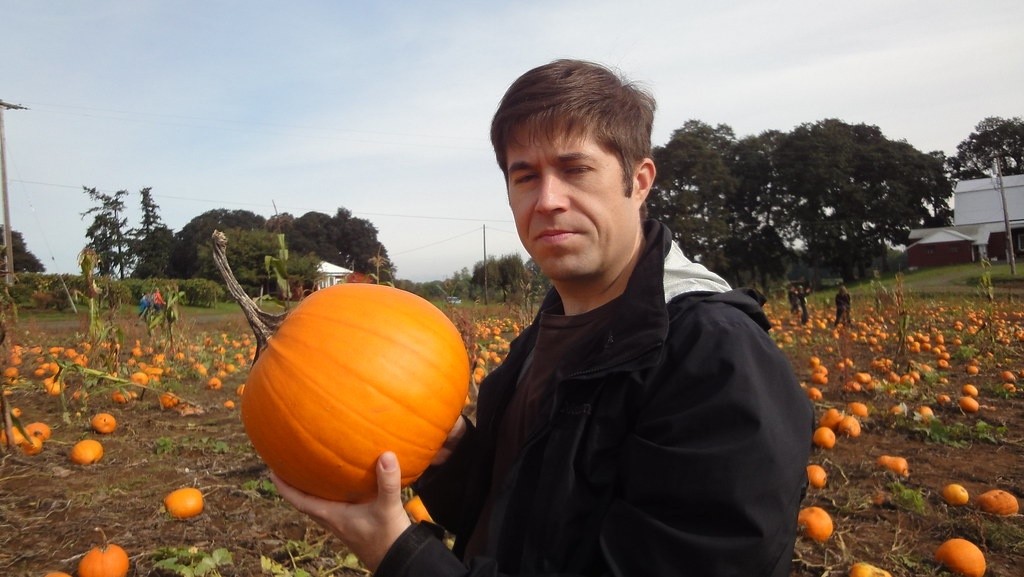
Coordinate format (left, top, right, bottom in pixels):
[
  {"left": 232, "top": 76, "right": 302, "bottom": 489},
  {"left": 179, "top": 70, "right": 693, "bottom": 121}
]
[{"left": 6, "top": 281, "right": 1024, "bottom": 577}]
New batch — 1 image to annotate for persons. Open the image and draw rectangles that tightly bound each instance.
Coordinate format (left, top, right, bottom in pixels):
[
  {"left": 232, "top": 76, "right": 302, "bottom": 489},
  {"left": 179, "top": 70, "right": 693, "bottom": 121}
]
[
  {"left": 151, "top": 288, "right": 166, "bottom": 314},
  {"left": 788, "top": 285, "right": 799, "bottom": 315},
  {"left": 139, "top": 291, "right": 150, "bottom": 322},
  {"left": 795, "top": 286, "right": 811, "bottom": 325},
  {"left": 835, "top": 285, "right": 851, "bottom": 327},
  {"left": 270, "top": 59, "right": 815, "bottom": 577}
]
